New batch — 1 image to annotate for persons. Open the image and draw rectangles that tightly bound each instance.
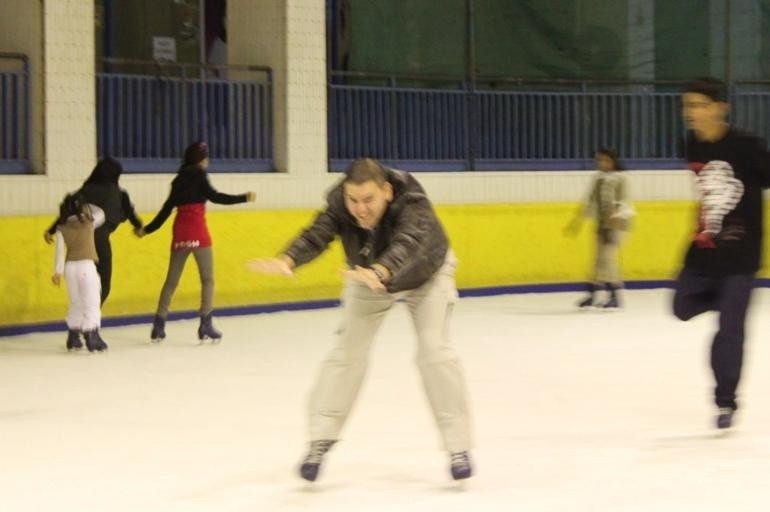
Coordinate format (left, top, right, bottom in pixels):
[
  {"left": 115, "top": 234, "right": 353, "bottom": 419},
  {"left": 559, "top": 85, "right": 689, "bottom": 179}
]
[
  {"left": 562, "top": 150, "right": 630, "bottom": 311},
  {"left": 672, "top": 76, "right": 770, "bottom": 438},
  {"left": 204, "top": 19, "right": 228, "bottom": 128},
  {"left": 134, "top": 141, "right": 257, "bottom": 347},
  {"left": 249, "top": 157, "right": 475, "bottom": 489},
  {"left": 52, "top": 192, "right": 108, "bottom": 354},
  {"left": 44, "top": 157, "right": 142, "bottom": 333}
]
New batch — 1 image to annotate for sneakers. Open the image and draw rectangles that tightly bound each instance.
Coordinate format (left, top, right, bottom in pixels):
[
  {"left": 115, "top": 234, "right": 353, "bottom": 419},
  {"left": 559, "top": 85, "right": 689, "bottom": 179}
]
[
  {"left": 604, "top": 297, "right": 618, "bottom": 308},
  {"left": 580, "top": 296, "right": 594, "bottom": 307}
]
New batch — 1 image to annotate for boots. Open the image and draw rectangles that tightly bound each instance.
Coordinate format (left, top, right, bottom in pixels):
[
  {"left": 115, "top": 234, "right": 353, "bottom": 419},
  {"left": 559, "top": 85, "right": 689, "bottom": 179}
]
[
  {"left": 67, "top": 329, "right": 82, "bottom": 347},
  {"left": 718, "top": 406, "right": 733, "bottom": 428},
  {"left": 198, "top": 310, "right": 221, "bottom": 338},
  {"left": 151, "top": 316, "right": 166, "bottom": 339},
  {"left": 84, "top": 328, "right": 107, "bottom": 349},
  {"left": 302, "top": 439, "right": 330, "bottom": 480},
  {"left": 450, "top": 451, "right": 470, "bottom": 479}
]
